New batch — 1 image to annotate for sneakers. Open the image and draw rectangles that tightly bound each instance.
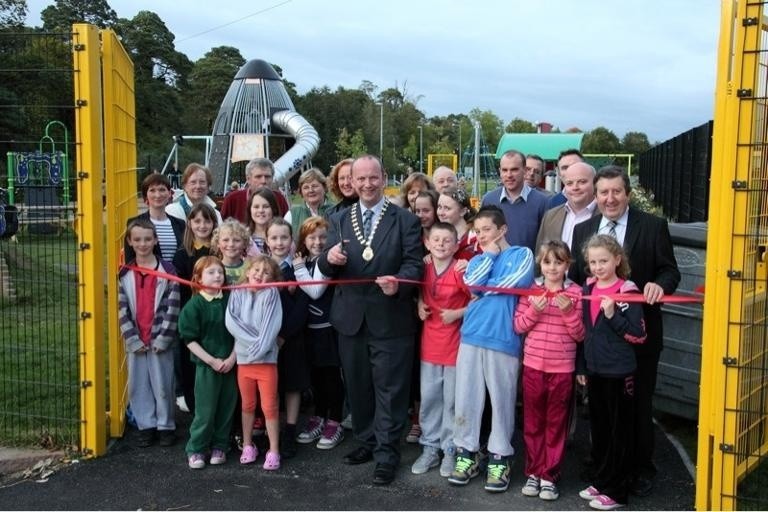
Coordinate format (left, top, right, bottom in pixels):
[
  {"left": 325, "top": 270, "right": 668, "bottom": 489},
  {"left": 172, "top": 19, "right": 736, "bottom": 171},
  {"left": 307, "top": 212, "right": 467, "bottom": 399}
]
[
  {"left": 630, "top": 478, "right": 656, "bottom": 497},
  {"left": 188, "top": 452, "right": 206, "bottom": 469},
  {"left": 159, "top": 428, "right": 176, "bottom": 447},
  {"left": 439, "top": 450, "right": 457, "bottom": 478},
  {"left": 520, "top": 474, "right": 541, "bottom": 497},
  {"left": 406, "top": 422, "right": 422, "bottom": 444},
  {"left": 588, "top": 494, "right": 627, "bottom": 510},
  {"left": 135, "top": 428, "right": 155, "bottom": 448},
  {"left": 537, "top": 477, "right": 561, "bottom": 501},
  {"left": 297, "top": 414, "right": 324, "bottom": 445},
  {"left": 578, "top": 485, "right": 599, "bottom": 499},
  {"left": 479, "top": 453, "right": 514, "bottom": 492},
  {"left": 209, "top": 448, "right": 227, "bottom": 465},
  {"left": 409, "top": 447, "right": 441, "bottom": 474},
  {"left": 314, "top": 417, "right": 346, "bottom": 452},
  {"left": 251, "top": 415, "right": 297, "bottom": 459}
]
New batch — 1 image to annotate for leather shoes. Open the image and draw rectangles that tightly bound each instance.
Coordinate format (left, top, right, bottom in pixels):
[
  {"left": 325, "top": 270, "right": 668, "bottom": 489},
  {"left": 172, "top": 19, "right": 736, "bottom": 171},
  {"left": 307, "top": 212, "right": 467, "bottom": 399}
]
[
  {"left": 371, "top": 461, "right": 397, "bottom": 486},
  {"left": 341, "top": 446, "right": 373, "bottom": 465},
  {"left": 447, "top": 452, "right": 480, "bottom": 485}
]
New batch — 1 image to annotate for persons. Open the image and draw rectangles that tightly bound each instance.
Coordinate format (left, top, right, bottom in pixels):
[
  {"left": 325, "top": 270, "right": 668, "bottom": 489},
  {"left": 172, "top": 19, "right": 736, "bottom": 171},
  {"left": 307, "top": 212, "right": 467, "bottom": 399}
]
[
  {"left": 397, "top": 148, "right": 683, "bottom": 510},
  {"left": 317, "top": 153, "right": 426, "bottom": 486},
  {"left": 116, "top": 157, "right": 357, "bottom": 473}
]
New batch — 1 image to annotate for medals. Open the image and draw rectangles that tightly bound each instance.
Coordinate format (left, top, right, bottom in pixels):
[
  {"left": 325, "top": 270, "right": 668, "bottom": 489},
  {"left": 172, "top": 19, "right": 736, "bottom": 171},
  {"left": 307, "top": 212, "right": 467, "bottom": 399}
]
[{"left": 360, "top": 245, "right": 376, "bottom": 262}]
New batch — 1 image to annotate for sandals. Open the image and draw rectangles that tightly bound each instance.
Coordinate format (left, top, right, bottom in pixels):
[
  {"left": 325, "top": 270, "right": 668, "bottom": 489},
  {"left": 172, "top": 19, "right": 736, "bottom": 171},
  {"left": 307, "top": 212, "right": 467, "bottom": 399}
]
[
  {"left": 263, "top": 448, "right": 281, "bottom": 471},
  {"left": 239, "top": 442, "right": 259, "bottom": 465}
]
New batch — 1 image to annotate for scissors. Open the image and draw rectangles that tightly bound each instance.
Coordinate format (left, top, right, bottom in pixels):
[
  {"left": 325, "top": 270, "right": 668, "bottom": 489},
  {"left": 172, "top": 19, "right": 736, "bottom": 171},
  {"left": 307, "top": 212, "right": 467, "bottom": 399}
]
[{"left": 337, "top": 219, "right": 351, "bottom": 263}]
